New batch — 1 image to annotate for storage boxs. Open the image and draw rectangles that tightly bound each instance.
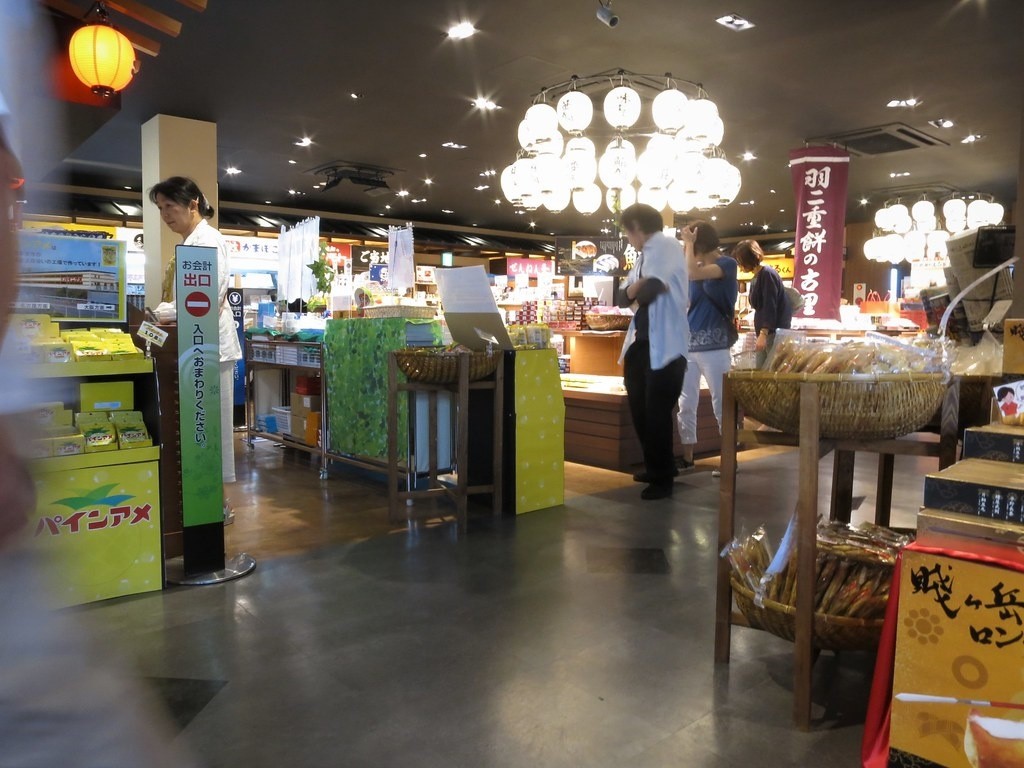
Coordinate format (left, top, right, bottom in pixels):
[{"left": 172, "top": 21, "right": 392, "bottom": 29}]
[
  {"left": 75, "top": 354, "right": 112, "bottom": 362},
  {"left": 305, "top": 411, "right": 322, "bottom": 448},
  {"left": 508, "top": 300, "right": 607, "bottom": 332},
  {"left": 44, "top": 424, "right": 85, "bottom": 456},
  {"left": 290, "top": 392, "right": 322, "bottom": 418},
  {"left": 290, "top": 415, "right": 307, "bottom": 440},
  {"left": 253, "top": 346, "right": 275, "bottom": 363},
  {"left": 119, "top": 436, "right": 152, "bottom": 450},
  {"left": 297, "top": 347, "right": 320, "bottom": 368},
  {"left": 73, "top": 380, "right": 134, "bottom": 413},
  {"left": 271, "top": 406, "right": 291, "bottom": 436},
  {"left": 111, "top": 347, "right": 144, "bottom": 361},
  {"left": 84, "top": 441, "right": 119, "bottom": 454},
  {"left": 275, "top": 346, "right": 297, "bottom": 366},
  {"left": 251, "top": 335, "right": 270, "bottom": 349},
  {"left": 30, "top": 431, "right": 54, "bottom": 459}
]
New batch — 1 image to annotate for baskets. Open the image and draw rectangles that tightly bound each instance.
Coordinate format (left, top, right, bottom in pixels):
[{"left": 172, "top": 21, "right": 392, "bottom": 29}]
[
  {"left": 585, "top": 314, "right": 634, "bottom": 330},
  {"left": 729, "top": 572, "right": 886, "bottom": 652},
  {"left": 391, "top": 344, "right": 502, "bottom": 384},
  {"left": 727, "top": 369, "right": 951, "bottom": 442}
]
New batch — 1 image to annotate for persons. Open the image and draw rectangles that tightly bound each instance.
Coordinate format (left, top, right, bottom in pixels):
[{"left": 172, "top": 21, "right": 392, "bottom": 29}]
[
  {"left": 674, "top": 221, "right": 744, "bottom": 475},
  {"left": 732, "top": 238, "right": 787, "bottom": 372},
  {"left": 144, "top": 175, "right": 242, "bottom": 528},
  {"left": 616, "top": 201, "right": 693, "bottom": 501}
]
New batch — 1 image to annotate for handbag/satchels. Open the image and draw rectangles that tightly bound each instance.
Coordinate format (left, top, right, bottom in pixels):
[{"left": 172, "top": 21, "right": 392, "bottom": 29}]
[{"left": 727, "top": 320, "right": 738, "bottom": 347}]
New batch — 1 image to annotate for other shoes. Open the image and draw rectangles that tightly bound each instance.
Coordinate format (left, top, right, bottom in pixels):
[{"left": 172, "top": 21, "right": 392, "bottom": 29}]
[
  {"left": 676, "top": 459, "right": 695, "bottom": 472},
  {"left": 639, "top": 483, "right": 673, "bottom": 500},
  {"left": 710, "top": 465, "right": 739, "bottom": 475},
  {"left": 632, "top": 465, "right": 680, "bottom": 482}
]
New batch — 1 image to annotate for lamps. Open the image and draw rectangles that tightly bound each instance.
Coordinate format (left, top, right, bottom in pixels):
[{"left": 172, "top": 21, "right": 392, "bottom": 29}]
[
  {"left": 69, "top": 0, "right": 136, "bottom": 98},
  {"left": 596, "top": 0, "right": 619, "bottom": 29},
  {"left": 863, "top": 182, "right": 1005, "bottom": 290},
  {"left": 500, "top": 67, "right": 742, "bottom": 218}
]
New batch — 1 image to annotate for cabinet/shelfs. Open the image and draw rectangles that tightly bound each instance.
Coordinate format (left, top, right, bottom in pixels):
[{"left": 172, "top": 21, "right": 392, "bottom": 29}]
[{"left": 25, "top": 359, "right": 162, "bottom": 610}]
